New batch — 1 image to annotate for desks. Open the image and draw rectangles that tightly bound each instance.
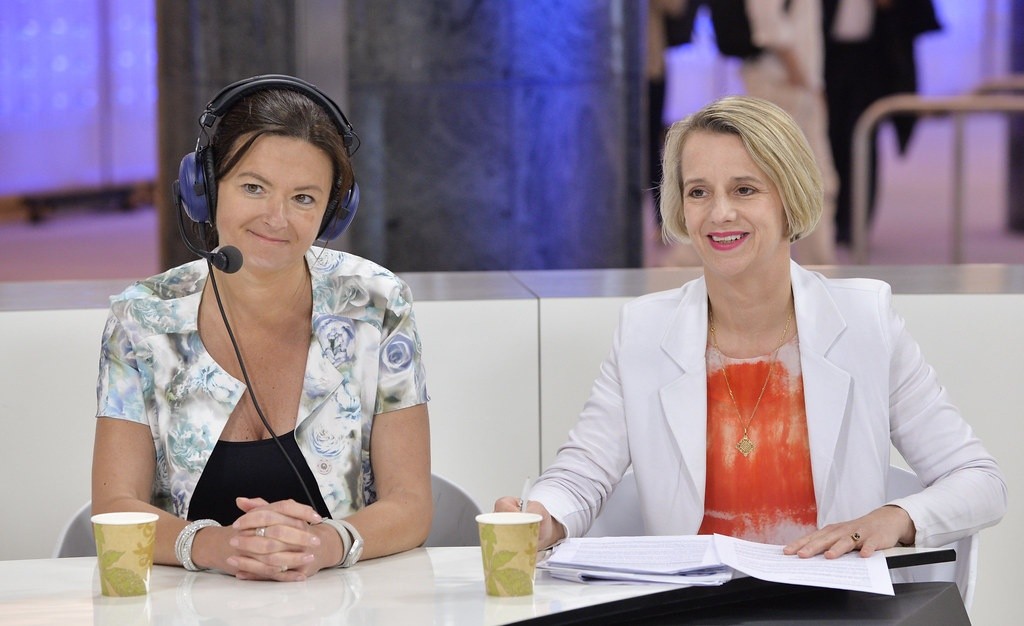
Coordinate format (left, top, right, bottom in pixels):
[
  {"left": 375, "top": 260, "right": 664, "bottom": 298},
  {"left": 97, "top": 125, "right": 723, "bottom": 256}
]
[{"left": 0, "top": 545, "right": 961, "bottom": 626}]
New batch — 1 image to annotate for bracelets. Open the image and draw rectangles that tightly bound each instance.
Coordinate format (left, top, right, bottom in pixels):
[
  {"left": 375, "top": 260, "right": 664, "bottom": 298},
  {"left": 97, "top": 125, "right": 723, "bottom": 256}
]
[
  {"left": 175, "top": 519, "right": 222, "bottom": 571},
  {"left": 310, "top": 517, "right": 351, "bottom": 566}
]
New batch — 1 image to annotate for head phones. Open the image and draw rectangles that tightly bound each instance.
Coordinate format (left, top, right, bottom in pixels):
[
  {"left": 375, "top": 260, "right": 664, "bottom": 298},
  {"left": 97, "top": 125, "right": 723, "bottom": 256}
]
[{"left": 178, "top": 74, "right": 360, "bottom": 243}]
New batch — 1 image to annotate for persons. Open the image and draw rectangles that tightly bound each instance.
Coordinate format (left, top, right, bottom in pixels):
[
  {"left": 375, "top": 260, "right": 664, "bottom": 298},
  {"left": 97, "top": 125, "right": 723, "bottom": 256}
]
[
  {"left": 91, "top": 85, "right": 435, "bottom": 581},
  {"left": 494, "top": 96, "right": 1009, "bottom": 584},
  {"left": 647, "top": 1, "right": 942, "bottom": 250}
]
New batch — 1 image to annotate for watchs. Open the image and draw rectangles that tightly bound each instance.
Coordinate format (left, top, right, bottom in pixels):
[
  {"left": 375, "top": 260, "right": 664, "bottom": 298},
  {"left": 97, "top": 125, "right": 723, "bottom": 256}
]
[{"left": 333, "top": 519, "right": 364, "bottom": 568}]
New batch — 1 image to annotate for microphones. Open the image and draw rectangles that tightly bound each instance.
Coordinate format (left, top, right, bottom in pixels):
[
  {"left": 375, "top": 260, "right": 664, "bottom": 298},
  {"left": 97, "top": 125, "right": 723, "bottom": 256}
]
[{"left": 172, "top": 179, "right": 243, "bottom": 275}]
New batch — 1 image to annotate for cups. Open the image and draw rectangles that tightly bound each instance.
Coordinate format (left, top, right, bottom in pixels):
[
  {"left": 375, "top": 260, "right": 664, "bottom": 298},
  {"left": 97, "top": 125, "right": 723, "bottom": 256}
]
[
  {"left": 90, "top": 511, "right": 159, "bottom": 597},
  {"left": 475, "top": 512, "right": 543, "bottom": 596}
]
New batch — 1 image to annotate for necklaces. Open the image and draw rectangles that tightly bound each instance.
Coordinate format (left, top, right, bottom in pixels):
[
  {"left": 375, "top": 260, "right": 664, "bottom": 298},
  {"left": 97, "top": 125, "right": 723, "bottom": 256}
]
[{"left": 709, "top": 308, "right": 793, "bottom": 457}]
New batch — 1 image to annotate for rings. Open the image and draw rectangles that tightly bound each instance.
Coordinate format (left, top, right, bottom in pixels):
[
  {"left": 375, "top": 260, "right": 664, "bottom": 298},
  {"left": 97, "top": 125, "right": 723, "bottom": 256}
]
[
  {"left": 849, "top": 533, "right": 860, "bottom": 546},
  {"left": 256, "top": 527, "right": 266, "bottom": 537}
]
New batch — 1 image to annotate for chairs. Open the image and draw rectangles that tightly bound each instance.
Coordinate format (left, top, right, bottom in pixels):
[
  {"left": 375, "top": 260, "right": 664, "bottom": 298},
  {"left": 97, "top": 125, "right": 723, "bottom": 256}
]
[
  {"left": 580, "top": 463, "right": 979, "bottom": 617},
  {"left": 54, "top": 469, "right": 481, "bottom": 557}
]
